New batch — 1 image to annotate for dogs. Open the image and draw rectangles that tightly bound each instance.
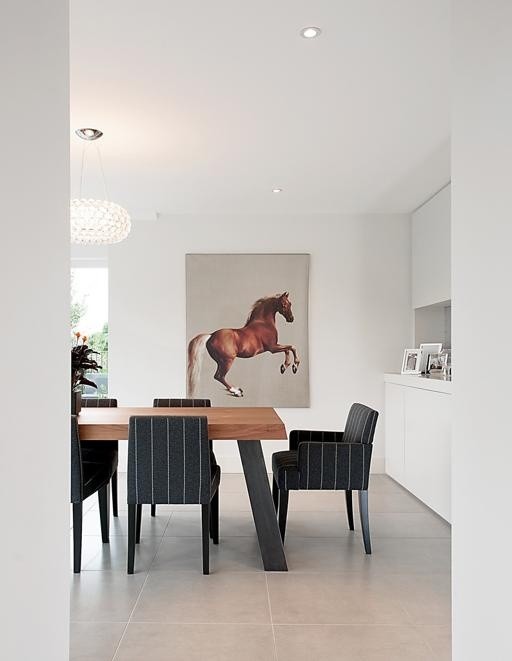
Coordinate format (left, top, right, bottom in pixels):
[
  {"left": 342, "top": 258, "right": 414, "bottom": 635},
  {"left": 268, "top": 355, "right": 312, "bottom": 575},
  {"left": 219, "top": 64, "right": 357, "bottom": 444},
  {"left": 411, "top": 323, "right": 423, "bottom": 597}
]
[{"left": 187, "top": 292, "right": 300, "bottom": 399}]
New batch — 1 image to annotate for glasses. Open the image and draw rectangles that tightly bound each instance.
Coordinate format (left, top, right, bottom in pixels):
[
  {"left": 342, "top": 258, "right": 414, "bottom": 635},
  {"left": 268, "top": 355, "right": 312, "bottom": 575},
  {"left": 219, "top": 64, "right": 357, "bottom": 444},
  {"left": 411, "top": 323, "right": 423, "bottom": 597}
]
[{"left": 70, "top": 128, "right": 132, "bottom": 247}]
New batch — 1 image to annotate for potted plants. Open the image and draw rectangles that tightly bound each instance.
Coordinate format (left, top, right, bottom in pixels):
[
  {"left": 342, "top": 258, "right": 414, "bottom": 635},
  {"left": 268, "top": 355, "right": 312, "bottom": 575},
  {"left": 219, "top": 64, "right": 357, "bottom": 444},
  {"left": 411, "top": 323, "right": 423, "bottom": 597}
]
[
  {"left": 420, "top": 343, "right": 443, "bottom": 373},
  {"left": 401, "top": 349, "right": 420, "bottom": 374}
]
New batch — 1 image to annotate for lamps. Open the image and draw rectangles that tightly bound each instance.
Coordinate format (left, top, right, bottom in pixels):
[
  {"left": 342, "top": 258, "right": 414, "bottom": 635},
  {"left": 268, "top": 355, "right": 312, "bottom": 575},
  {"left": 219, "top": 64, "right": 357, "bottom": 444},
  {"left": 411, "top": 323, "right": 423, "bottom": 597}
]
[
  {"left": 151, "top": 398, "right": 216, "bottom": 517},
  {"left": 128, "top": 415, "right": 220, "bottom": 575},
  {"left": 82, "top": 399, "right": 118, "bottom": 516},
  {"left": 70, "top": 415, "right": 110, "bottom": 573},
  {"left": 272, "top": 403, "right": 379, "bottom": 554}
]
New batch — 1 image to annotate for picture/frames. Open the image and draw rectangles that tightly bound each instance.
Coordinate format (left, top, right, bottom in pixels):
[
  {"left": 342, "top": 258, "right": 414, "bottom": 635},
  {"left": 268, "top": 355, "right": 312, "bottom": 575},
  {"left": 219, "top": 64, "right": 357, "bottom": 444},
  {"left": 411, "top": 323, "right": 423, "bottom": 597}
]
[{"left": 75, "top": 407, "right": 289, "bottom": 571}]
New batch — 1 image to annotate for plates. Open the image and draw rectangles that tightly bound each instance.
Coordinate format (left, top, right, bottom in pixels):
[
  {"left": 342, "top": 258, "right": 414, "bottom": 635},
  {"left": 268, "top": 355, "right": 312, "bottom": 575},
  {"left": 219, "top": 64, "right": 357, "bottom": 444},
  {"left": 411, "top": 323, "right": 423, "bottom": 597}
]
[{"left": 385, "top": 383, "right": 451, "bottom": 524}]
